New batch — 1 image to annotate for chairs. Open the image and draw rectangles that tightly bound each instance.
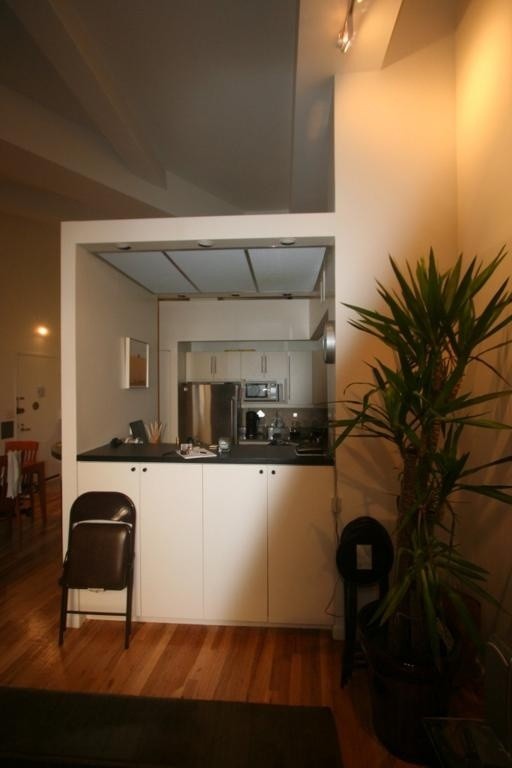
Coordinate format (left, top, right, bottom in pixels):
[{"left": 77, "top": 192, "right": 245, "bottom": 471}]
[
  {"left": 56, "top": 492, "right": 134, "bottom": 650},
  {"left": 1, "top": 440, "right": 49, "bottom": 544}
]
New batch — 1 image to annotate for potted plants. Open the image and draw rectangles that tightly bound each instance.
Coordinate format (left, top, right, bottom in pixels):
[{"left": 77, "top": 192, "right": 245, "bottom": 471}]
[{"left": 319, "top": 243, "right": 511, "bottom": 766}]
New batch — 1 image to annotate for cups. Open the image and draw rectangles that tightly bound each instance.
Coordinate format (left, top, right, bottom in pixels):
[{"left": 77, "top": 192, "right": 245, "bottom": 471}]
[
  {"left": 144, "top": 422, "right": 168, "bottom": 444},
  {"left": 179, "top": 436, "right": 234, "bottom": 455}
]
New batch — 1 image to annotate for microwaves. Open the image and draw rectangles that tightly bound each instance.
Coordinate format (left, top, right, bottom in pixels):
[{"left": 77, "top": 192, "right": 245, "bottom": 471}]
[{"left": 244, "top": 382, "right": 278, "bottom": 402}]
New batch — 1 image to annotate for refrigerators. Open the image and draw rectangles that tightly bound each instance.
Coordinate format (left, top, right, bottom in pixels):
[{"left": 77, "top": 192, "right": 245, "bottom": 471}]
[{"left": 177, "top": 383, "right": 239, "bottom": 445}]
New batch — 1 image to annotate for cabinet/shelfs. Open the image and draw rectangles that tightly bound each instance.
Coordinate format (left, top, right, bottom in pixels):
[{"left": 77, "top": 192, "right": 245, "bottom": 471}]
[
  {"left": 76, "top": 460, "right": 341, "bottom": 631},
  {"left": 185, "top": 351, "right": 288, "bottom": 382},
  {"left": 289, "top": 350, "right": 326, "bottom": 405}
]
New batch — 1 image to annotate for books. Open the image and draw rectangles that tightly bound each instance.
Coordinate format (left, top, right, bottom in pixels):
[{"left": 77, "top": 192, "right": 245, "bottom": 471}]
[{"left": 175, "top": 444, "right": 218, "bottom": 462}]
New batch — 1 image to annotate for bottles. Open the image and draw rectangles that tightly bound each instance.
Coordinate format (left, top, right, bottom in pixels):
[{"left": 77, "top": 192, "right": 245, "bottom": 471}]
[{"left": 240, "top": 409, "right": 299, "bottom": 445}]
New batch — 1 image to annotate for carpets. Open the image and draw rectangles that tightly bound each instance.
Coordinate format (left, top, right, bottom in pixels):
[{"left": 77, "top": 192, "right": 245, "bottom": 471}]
[{"left": 0, "top": 686, "right": 341, "bottom": 767}]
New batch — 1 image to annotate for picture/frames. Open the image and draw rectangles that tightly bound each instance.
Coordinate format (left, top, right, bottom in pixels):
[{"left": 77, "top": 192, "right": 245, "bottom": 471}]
[{"left": 122, "top": 335, "right": 152, "bottom": 391}]
